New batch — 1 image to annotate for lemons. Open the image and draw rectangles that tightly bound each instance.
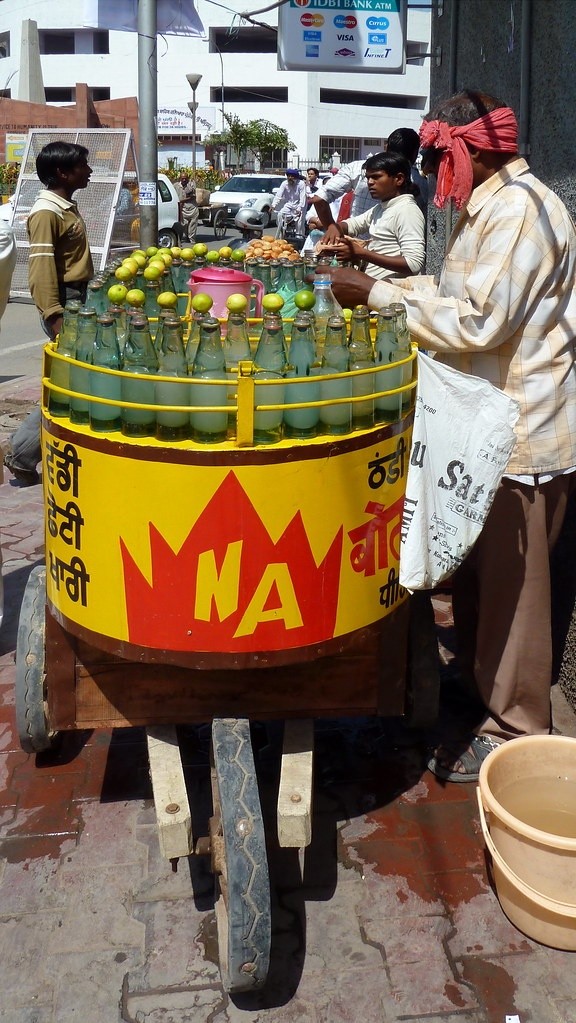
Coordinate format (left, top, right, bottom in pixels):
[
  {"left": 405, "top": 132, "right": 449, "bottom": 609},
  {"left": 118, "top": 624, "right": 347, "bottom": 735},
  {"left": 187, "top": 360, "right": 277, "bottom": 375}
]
[
  {"left": 262, "top": 293, "right": 284, "bottom": 311},
  {"left": 294, "top": 290, "right": 316, "bottom": 310},
  {"left": 192, "top": 293, "right": 213, "bottom": 313},
  {"left": 226, "top": 293, "right": 248, "bottom": 313},
  {"left": 114, "top": 243, "right": 246, "bottom": 279},
  {"left": 157, "top": 291, "right": 178, "bottom": 308},
  {"left": 342, "top": 305, "right": 364, "bottom": 320},
  {"left": 108, "top": 284, "right": 146, "bottom": 306}
]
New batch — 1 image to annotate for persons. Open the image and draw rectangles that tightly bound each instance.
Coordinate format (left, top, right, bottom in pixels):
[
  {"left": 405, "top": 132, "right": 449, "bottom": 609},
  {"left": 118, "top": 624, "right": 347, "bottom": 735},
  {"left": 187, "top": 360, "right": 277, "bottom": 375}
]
[
  {"left": 0, "top": 142, "right": 92, "bottom": 483},
  {"left": 116, "top": 179, "right": 139, "bottom": 243},
  {"left": 269, "top": 169, "right": 308, "bottom": 240},
  {"left": 162, "top": 172, "right": 200, "bottom": 243},
  {"left": 307, "top": 92, "right": 576, "bottom": 781},
  {"left": 306, "top": 128, "right": 427, "bottom": 282},
  {"left": 305, "top": 168, "right": 322, "bottom": 194}
]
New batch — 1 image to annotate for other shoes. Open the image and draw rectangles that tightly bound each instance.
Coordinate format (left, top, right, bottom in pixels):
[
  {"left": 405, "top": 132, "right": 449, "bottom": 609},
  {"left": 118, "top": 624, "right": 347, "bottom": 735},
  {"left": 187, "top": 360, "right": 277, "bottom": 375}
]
[
  {"left": 0, "top": 439, "right": 39, "bottom": 484},
  {"left": 190, "top": 237, "right": 197, "bottom": 243}
]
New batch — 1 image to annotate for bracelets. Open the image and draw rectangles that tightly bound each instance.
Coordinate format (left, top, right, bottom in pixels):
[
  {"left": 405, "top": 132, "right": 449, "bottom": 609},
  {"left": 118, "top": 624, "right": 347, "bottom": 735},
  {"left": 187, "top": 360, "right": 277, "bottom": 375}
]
[{"left": 317, "top": 218, "right": 319, "bottom": 224}]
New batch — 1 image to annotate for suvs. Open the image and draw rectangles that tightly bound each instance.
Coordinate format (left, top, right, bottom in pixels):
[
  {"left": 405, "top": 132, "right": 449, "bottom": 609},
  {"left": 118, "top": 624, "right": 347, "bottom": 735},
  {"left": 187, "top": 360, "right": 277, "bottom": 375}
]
[{"left": 0, "top": 169, "right": 184, "bottom": 254}]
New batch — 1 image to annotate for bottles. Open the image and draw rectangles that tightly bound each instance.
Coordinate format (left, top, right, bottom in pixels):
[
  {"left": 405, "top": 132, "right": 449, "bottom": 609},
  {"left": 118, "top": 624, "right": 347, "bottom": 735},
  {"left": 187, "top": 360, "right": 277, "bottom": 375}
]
[{"left": 47, "top": 248, "right": 418, "bottom": 443}]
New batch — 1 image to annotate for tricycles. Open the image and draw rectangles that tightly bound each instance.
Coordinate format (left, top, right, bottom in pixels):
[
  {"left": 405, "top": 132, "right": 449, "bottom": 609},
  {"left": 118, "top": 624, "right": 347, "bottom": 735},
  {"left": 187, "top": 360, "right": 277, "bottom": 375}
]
[
  {"left": 16, "top": 562, "right": 448, "bottom": 994},
  {"left": 181, "top": 199, "right": 228, "bottom": 241}
]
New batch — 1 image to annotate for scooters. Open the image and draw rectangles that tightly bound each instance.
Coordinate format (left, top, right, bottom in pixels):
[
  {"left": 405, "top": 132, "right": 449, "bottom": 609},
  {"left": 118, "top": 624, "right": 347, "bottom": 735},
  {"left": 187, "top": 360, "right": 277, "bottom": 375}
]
[{"left": 225, "top": 208, "right": 305, "bottom": 255}]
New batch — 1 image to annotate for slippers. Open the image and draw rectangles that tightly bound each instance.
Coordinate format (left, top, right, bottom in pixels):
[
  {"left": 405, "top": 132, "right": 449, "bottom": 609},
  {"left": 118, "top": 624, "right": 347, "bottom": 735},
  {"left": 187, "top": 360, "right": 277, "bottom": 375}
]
[{"left": 425, "top": 721, "right": 502, "bottom": 783}]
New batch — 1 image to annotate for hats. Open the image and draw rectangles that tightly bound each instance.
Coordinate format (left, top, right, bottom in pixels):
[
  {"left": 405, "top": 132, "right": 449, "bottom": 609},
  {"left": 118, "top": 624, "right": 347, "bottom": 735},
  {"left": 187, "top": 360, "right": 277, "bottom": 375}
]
[
  {"left": 286, "top": 169, "right": 299, "bottom": 176},
  {"left": 330, "top": 168, "right": 339, "bottom": 173}
]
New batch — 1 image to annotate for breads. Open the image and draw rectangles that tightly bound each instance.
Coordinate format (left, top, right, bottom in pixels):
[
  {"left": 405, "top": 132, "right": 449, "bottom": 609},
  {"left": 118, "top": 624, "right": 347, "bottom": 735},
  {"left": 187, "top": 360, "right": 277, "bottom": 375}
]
[{"left": 244, "top": 236, "right": 301, "bottom": 261}]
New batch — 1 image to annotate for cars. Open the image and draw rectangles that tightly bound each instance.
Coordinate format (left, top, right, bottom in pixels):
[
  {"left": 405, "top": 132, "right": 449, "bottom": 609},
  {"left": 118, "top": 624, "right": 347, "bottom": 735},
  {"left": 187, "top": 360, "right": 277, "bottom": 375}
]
[{"left": 202, "top": 172, "right": 287, "bottom": 228}]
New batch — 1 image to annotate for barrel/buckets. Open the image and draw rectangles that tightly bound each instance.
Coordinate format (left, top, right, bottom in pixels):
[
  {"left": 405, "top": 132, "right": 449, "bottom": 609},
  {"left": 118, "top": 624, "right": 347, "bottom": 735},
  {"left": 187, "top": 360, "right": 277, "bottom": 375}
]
[{"left": 476, "top": 733, "right": 576, "bottom": 952}]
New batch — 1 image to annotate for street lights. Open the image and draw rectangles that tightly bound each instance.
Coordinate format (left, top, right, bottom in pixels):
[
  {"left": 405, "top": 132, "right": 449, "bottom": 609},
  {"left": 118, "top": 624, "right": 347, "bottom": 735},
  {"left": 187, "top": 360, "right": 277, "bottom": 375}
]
[{"left": 184, "top": 72, "right": 203, "bottom": 181}]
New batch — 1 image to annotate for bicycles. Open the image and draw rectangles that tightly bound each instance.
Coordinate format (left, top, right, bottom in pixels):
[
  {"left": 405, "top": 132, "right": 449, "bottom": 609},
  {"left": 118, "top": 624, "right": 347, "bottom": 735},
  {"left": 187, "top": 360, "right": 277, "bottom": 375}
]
[{"left": 269, "top": 208, "right": 307, "bottom": 254}]
